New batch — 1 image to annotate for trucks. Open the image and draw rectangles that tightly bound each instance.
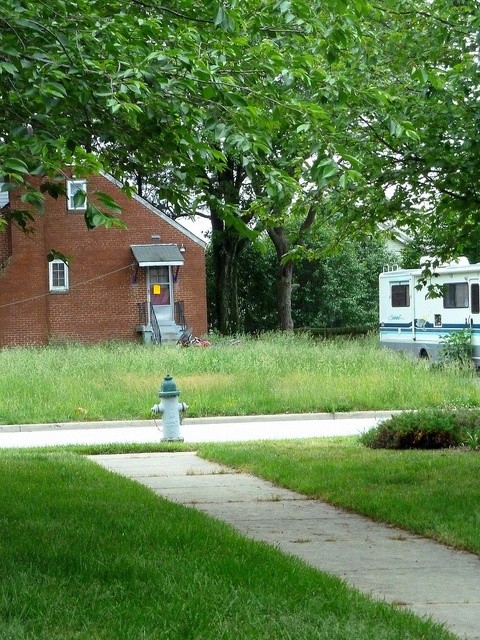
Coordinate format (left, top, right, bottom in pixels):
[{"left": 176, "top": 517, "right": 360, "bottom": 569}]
[{"left": 379, "top": 255, "right": 480, "bottom": 371}]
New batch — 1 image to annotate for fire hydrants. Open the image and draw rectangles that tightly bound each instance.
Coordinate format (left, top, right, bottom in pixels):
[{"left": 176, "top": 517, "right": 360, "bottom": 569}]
[{"left": 150, "top": 374, "right": 189, "bottom": 444}]
